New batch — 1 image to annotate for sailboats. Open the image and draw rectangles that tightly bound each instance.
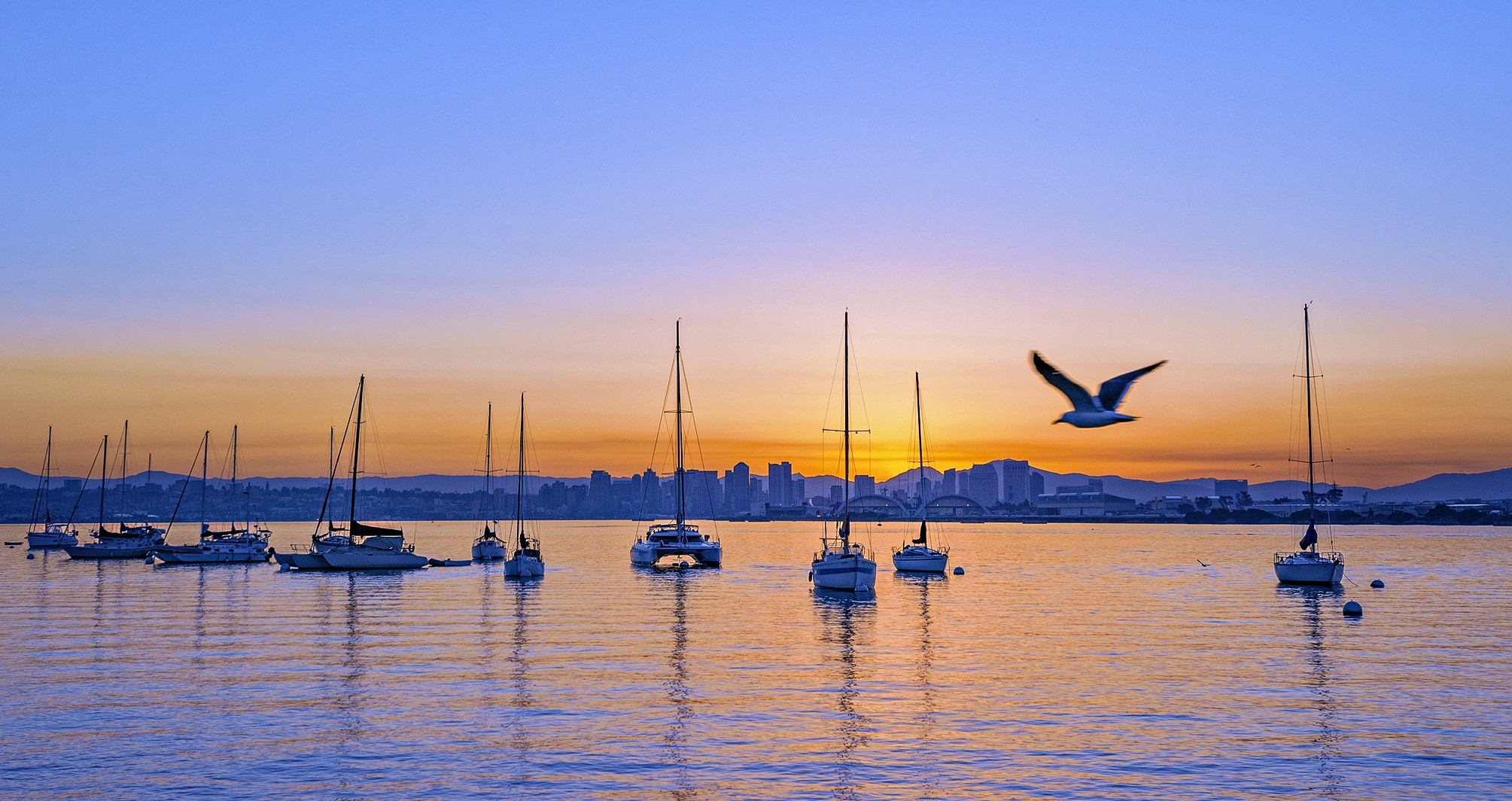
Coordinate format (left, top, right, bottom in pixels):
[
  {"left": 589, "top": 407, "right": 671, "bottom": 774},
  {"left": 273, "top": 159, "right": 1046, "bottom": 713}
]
[
  {"left": 25, "top": 425, "right": 80, "bottom": 546},
  {"left": 151, "top": 430, "right": 267, "bottom": 563},
  {"left": 470, "top": 401, "right": 510, "bottom": 560},
  {"left": 93, "top": 419, "right": 164, "bottom": 547},
  {"left": 279, "top": 372, "right": 426, "bottom": 571},
  {"left": 1269, "top": 303, "right": 1346, "bottom": 587},
  {"left": 892, "top": 366, "right": 949, "bottom": 575},
  {"left": 500, "top": 391, "right": 545, "bottom": 579},
  {"left": 118, "top": 452, "right": 167, "bottom": 537},
  {"left": 808, "top": 306, "right": 878, "bottom": 595},
  {"left": 49, "top": 434, "right": 154, "bottom": 557},
  {"left": 202, "top": 424, "right": 270, "bottom": 550},
  {"left": 628, "top": 319, "right": 721, "bottom": 571}
]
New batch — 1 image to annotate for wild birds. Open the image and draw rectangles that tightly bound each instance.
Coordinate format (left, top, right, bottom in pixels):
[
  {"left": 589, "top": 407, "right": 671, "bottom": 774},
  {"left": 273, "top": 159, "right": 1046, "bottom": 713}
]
[
  {"left": 1028, "top": 348, "right": 1169, "bottom": 428},
  {"left": 1197, "top": 559, "right": 1212, "bottom": 568}
]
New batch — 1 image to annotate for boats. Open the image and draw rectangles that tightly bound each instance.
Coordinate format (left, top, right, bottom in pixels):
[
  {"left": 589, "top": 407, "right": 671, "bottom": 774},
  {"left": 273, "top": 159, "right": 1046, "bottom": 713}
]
[{"left": 433, "top": 558, "right": 472, "bottom": 568}]
